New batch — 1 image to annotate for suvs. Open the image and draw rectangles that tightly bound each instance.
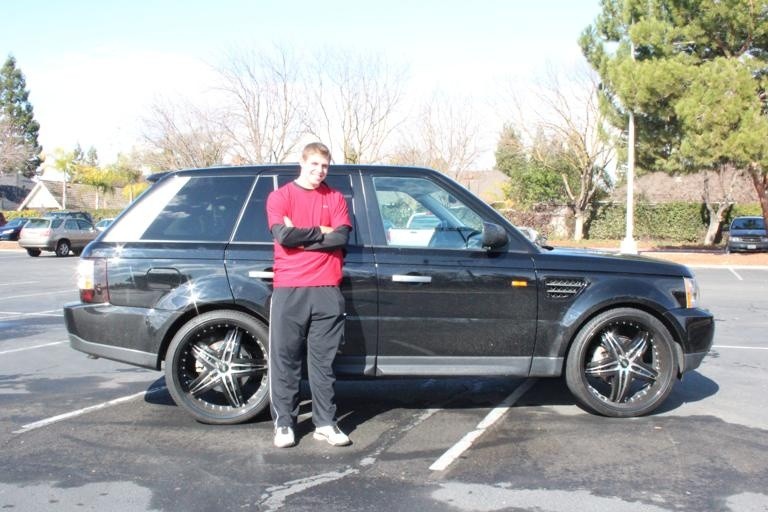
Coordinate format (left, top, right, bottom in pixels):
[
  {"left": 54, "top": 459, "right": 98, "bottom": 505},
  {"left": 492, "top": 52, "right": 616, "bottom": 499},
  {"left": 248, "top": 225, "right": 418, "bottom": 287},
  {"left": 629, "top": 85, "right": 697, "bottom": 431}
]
[
  {"left": 64, "top": 158, "right": 716, "bottom": 421},
  {"left": 0, "top": 207, "right": 115, "bottom": 257}
]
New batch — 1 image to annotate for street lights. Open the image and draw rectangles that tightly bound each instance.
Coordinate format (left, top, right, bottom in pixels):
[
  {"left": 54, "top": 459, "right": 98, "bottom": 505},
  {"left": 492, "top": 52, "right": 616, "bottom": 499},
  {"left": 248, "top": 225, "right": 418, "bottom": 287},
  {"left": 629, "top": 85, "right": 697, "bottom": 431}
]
[{"left": 602, "top": 34, "right": 640, "bottom": 256}]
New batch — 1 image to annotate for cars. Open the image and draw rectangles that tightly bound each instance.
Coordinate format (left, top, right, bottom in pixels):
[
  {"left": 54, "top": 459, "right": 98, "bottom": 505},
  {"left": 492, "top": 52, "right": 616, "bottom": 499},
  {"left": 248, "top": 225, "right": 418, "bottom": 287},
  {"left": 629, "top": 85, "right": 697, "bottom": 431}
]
[
  {"left": 727, "top": 216, "right": 768, "bottom": 254},
  {"left": 386, "top": 199, "right": 517, "bottom": 245}
]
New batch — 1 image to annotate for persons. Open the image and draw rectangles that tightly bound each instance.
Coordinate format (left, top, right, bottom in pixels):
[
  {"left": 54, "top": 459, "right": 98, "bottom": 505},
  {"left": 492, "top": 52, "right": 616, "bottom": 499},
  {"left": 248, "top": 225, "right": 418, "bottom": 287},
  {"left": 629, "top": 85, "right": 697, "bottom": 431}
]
[{"left": 265, "top": 143, "right": 353, "bottom": 449}]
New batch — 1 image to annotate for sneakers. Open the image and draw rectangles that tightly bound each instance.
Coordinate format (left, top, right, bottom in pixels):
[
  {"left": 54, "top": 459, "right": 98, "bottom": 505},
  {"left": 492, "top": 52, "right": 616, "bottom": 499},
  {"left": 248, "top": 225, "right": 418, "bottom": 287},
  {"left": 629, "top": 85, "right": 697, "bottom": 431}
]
[
  {"left": 272, "top": 424, "right": 298, "bottom": 448},
  {"left": 312, "top": 424, "right": 352, "bottom": 447}
]
